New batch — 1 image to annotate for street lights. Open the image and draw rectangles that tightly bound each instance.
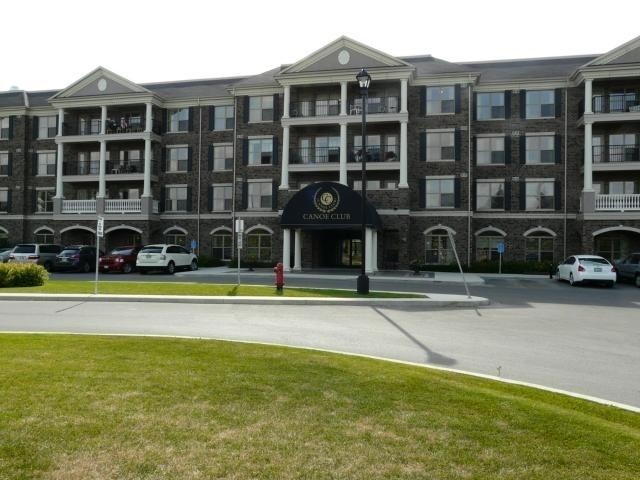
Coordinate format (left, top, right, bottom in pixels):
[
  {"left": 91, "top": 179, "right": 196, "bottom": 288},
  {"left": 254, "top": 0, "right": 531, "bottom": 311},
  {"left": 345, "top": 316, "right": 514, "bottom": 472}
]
[{"left": 354, "top": 66, "right": 373, "bottom": 294}]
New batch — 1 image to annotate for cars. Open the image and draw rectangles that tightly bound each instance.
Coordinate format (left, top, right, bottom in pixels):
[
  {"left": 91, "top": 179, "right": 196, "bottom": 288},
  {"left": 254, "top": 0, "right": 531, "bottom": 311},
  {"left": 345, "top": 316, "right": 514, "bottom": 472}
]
[
  {"left": 555, "top": 254, "right": 617, "bottom": 287},
  {"left": 0, "top": 248, "right": 13, "bottom": 260},
  {"left": 615, "top": 252, "right": 640, "bottom": 288},
  {"left": 98, "top": 244, "right": 143, "bottom": 273}
]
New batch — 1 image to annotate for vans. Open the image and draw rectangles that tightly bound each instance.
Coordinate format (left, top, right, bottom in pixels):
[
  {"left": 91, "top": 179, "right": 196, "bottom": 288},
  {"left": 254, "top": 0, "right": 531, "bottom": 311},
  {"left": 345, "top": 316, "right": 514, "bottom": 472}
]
[{"left": 8, "top": 243, "right": 64, "bottom": 272}]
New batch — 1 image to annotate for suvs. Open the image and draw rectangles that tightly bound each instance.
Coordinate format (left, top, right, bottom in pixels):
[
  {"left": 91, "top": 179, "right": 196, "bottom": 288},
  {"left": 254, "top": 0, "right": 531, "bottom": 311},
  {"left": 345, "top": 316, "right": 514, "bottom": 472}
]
[
  {"left": 135, "top": 243, "right": 199, "bottom": 274},
  {"left": 56, "top": 244, "right": 105, "bottom": 272}
]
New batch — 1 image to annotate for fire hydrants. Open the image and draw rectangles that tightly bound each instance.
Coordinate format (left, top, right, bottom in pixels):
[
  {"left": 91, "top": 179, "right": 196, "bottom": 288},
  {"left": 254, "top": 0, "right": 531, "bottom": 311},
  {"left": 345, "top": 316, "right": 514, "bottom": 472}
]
[{"left": 274, "top": 262, "right": 285, "bottom": 289}]
